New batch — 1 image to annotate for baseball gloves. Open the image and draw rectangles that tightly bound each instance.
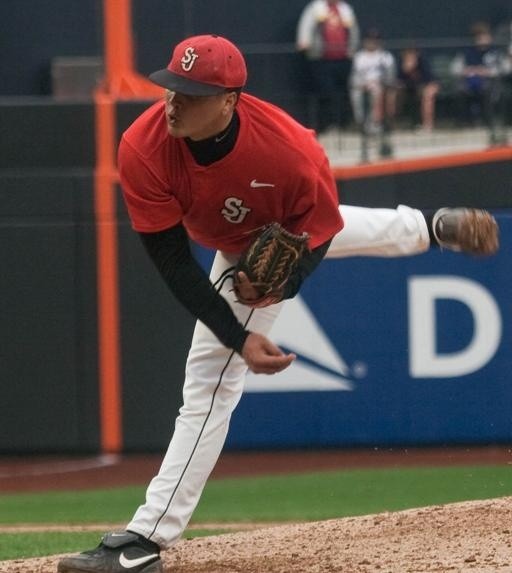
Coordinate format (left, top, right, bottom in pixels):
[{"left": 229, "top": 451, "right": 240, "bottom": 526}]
[{"left": 234, "top": 223, "right": 307, "bottom": 307}]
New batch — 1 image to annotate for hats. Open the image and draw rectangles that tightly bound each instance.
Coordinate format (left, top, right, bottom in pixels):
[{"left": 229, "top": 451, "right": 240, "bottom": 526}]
[{"left": 148, "top": 31, "right": 247, "bottom": 99}]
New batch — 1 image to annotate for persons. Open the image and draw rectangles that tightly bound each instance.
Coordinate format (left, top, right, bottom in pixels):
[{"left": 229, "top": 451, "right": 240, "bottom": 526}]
[
  {"left": 296, "top": 0, "right": 360, "bottom": 134},
  {"left": 57, "top": 32, "right": 501, "bottom": 573},
  {"left": 388, "top": 49, "right": 446, "bottom": 136},
  {"left": 348, "top": 24, "right": 396, "bottom": 138},
  {"left": 449, "top": 21, "right": 511, "bottom": 135}
]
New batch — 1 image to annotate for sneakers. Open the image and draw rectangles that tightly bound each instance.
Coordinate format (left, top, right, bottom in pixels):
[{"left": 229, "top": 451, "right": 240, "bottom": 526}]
[
  {"left": 56, "top": 527, "right": 162, "bottom": 573},
  {"left": 433, "top": 204, "right": 500, "bottom": 256}
]
